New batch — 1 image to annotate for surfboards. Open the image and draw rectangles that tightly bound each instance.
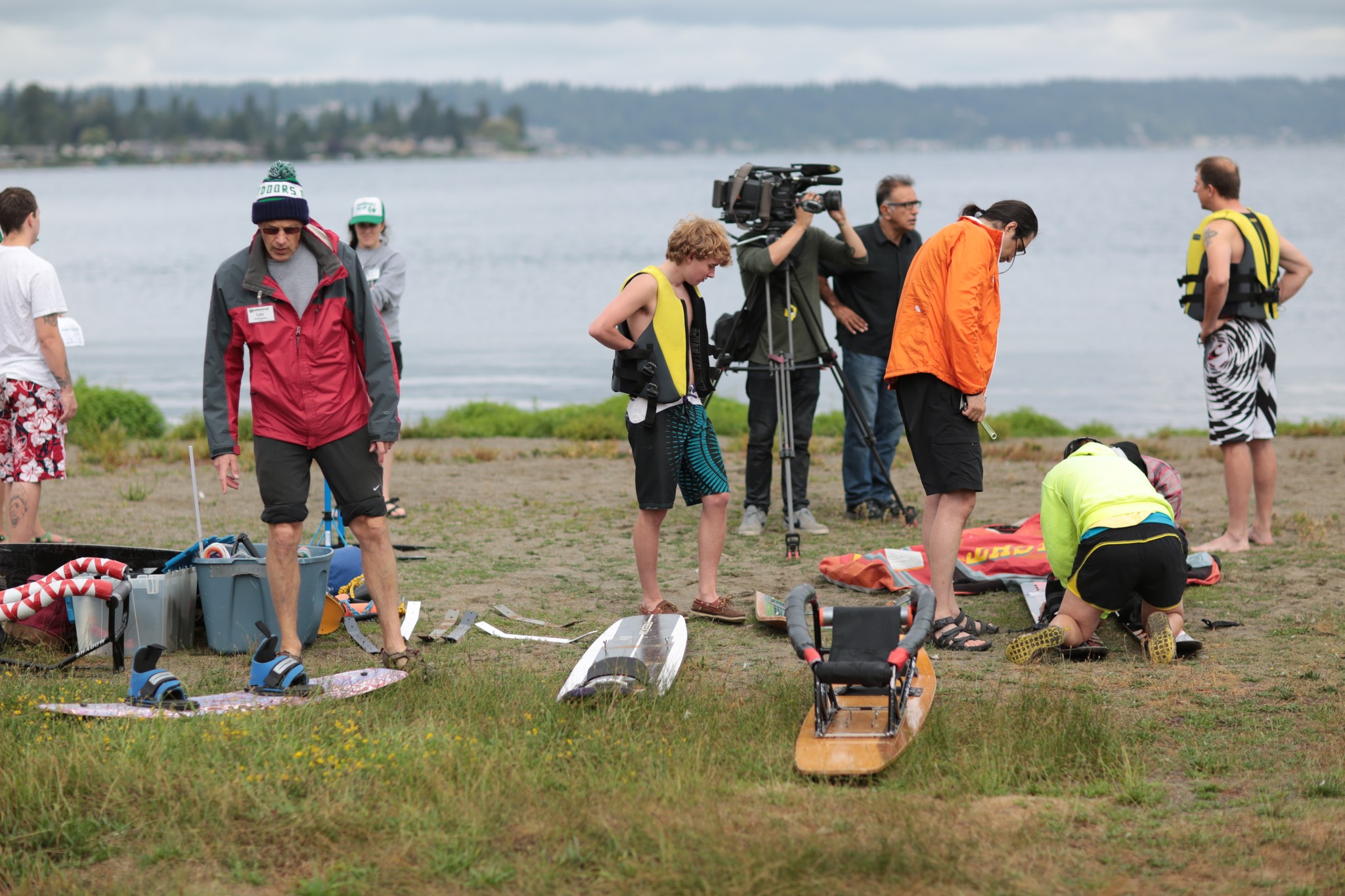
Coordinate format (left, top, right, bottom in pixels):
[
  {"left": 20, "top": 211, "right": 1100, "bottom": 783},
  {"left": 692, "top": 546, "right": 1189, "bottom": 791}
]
[
  {"left": 1020, "top": 579, "right": 1202, "bottom": 655},
  {"left": 555, "top": 614, "right": 687, "bottom": 702},
  {"left": 38, "top": 667, "right": 409, "bottom": 718}
]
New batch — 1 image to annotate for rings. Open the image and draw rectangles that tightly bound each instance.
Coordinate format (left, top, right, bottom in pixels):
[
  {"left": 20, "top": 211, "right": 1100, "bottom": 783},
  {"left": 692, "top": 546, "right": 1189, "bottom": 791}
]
[{"left": 388, "top": 446, "right": 391, "bottom": 449}]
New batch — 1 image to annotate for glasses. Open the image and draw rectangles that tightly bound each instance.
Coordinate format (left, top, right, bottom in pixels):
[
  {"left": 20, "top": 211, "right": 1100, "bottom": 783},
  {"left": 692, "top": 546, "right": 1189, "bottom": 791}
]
[
  {"left": 260, "top": 222, "right": 303, "bottom": 235},
  {"left": 887, "top": 200, "right": 921, "bottom": 210},
  {"left": 1012, "top": 219, "right": 1026, "bottom": 256}
]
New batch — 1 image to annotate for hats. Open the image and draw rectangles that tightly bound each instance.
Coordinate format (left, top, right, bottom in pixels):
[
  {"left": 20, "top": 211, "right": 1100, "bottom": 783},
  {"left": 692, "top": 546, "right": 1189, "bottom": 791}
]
[
  {"left": 350, "top": 196, "right": 385, "bottom": 225},
  {"left": 251, "top": 160, "right": 309, "bottom": 225}
]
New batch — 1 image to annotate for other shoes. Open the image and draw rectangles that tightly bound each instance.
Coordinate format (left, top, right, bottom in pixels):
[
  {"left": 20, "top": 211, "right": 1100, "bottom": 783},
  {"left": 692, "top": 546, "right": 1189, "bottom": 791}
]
[
  {"left": 878, "top": 500, "right": 918, "bottom": 523},
  {"left": 1005, "top": 625, "right": 1070, "bottom": 665},
  {"left": 845, "top": 500, "right": 892, "bottom": 521},
  {"left": 1147, "top": 611, "right": 1177, "bottom": 664}
]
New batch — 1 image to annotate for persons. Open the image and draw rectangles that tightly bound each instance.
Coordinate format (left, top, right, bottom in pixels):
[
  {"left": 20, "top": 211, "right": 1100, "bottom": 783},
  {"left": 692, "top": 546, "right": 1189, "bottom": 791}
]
[
  {"left": 817, "top": 175, "right": 923, "bottom": 521},
  {"left": 201, "top": 160, "right": 435, "bottom": 681},
  {"left": 1177, "top": 157, "right": 1314, "bottom": 554},
  {"left": 0, "top": 187, "right": 79, "bottom": 546},
  {"left": 1004, "top": 438, "right": 1189, "bottom": 664},
  {"left": 330, "top": 195, "right": 407, "bottom": 519},
  {"left": 1110, "top": 441, "right": 1183, "bottom": 522},
  {"left": 587, "top": 216, "right": 749, "bottom": 622},
  {"left": 883, "top": 200, "right": 1039, "bottom": 651},
  {"left": 736, "top": 192, "right": 869, "bottom": 537}
]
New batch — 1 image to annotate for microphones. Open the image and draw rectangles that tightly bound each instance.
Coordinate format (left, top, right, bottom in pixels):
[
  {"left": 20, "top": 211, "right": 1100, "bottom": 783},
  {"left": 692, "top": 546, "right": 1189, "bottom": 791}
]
[
  {"left": 766, "top": 164, "right": 840, "bottom": 174},
  {"left": 818, "top": 177, "right": 842, "bottom": 184}
]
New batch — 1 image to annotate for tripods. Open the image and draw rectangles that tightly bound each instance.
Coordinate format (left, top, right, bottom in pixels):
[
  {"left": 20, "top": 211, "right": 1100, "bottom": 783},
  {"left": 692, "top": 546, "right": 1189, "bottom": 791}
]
[{"left": 701, "top": 237, "right": 916, "bottom": 558}]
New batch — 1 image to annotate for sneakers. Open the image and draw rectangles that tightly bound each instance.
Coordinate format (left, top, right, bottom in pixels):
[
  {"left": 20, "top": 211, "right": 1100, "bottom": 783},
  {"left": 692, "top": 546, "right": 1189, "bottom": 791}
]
[
  {"left": 690, "top": 594, "right": 746, "bottom": 622},
  {"left": 639, "top": 600, "right": 690, "bottom": 620},
  {"left": 784, "top": 507, "right": 829, "bottom": 535},
  {"left": 738, "top": 504, "right": 766, "bottom": 536}
]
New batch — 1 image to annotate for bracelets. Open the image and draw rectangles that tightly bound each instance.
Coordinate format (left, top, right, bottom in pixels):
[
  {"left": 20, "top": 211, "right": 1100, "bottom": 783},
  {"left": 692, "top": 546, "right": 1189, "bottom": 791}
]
[{"left": 63, "top": 392, "right": 74, "bottom": 398}]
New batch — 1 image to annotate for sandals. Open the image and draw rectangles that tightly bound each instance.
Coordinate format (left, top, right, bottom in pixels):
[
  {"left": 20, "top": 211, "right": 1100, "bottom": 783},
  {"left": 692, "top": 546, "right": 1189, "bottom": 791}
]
[
  {"left": 380, "top": 647, "right": 438, "bottom": 682},
  {"left": 331, "top": 506, "right": 338, "bottom": 520},
  {"left": 0, "top": 534, "right": 9, "bottom": 544},
  {"left": 953, "top": 607, "right": 999, "bottom": 637},
  {"left": 31, "top": 532, "right": 75, "bottom": 543},
  {"left": 123, "top": 643, "right": 198, "bottom": 710},
  {"left": 275, "top": 650, "right": 302, "bottom": 666},
  {"left": 925, "top": 616, "right": 992, "bottom": 652},
  {"left": 385, "top": 497, "right": 406, "bottom": 518},
  {"left": 244, "top": 620, "right": 325, "bottom": 698}
]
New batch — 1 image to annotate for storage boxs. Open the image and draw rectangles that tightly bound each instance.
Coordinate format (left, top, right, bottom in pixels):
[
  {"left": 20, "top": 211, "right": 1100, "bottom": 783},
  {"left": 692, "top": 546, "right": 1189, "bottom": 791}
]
[{"left": 73, "top": 544, "right": 334, "bottom": 659}]
